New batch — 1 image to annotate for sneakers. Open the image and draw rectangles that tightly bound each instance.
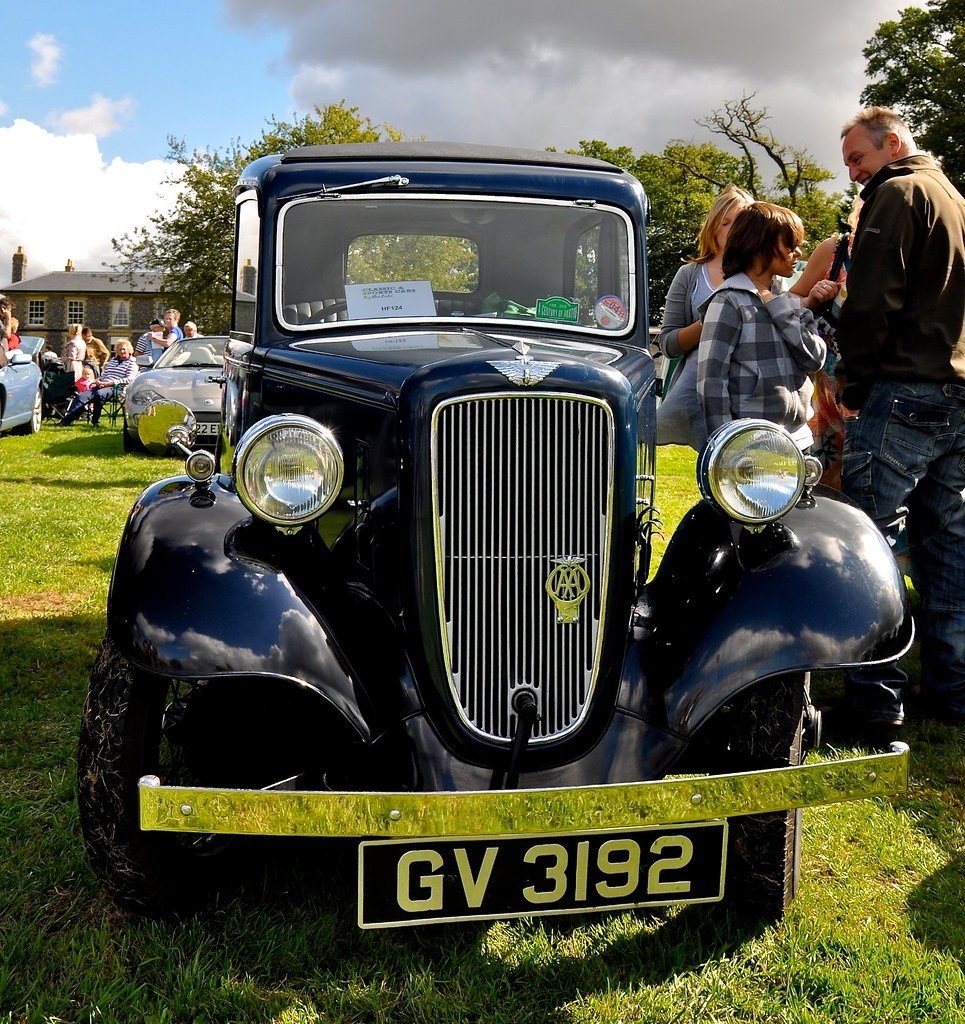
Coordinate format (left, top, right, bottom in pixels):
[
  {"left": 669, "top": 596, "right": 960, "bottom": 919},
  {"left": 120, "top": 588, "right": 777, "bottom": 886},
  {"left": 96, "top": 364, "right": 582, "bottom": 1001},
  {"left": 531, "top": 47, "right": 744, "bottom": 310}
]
[{"left": 824, "top": 703, "right": 903, "bottom": 753}]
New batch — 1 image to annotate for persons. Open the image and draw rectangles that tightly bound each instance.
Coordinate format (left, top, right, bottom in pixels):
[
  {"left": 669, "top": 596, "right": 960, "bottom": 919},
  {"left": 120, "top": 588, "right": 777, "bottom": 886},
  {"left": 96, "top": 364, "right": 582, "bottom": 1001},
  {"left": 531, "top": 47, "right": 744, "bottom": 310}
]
[
  {"left": 0, "top": 299, "right": 21, "bottom": 369},
  {"left": 655, "top": 106, "right": 965, "bottom": 751},
  {"left": 41, "top": 309, "right": 205, "bottom": 427}
]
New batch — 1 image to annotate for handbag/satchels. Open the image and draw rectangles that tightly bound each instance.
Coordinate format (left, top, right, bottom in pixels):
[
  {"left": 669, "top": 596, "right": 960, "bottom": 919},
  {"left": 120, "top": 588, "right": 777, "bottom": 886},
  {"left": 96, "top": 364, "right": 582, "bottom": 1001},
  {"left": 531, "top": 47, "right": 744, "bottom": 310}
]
[
  {"left": 808, "top": 301, "right": 834, "bottom": 331},
  {"left": 657, "top": 258, "right": 705, "bottom": 402}
]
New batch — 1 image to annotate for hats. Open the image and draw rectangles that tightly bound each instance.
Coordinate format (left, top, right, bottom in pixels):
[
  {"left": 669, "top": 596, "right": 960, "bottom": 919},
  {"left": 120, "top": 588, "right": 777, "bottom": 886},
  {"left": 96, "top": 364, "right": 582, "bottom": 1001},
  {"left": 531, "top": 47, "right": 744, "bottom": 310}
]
[
  {"left": 148, "top": 318, "right": 167, "bottom": 327},
  {"left": 82, "top": 328, "right": 91, "bottom": 334}
]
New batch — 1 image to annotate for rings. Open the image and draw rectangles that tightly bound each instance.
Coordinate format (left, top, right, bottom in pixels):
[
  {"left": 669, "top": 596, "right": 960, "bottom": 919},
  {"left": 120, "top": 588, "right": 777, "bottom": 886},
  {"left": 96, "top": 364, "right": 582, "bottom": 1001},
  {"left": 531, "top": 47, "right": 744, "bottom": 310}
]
[{"left": 824, "top": 284, "right": 827, "bottom": 288}]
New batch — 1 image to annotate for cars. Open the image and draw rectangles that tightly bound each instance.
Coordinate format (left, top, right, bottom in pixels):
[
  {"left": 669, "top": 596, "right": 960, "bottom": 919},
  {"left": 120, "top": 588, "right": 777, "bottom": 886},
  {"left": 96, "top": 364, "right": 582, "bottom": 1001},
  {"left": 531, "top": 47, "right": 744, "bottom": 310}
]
[
  {"left": 78, "top": 142, "right": 921, "bottom": 951},
  {"left": 124, "top": 335, "right": 230, "bottom": 452},
  {"left": 0, "top": 335, "right": 45, "bottom": 435}
]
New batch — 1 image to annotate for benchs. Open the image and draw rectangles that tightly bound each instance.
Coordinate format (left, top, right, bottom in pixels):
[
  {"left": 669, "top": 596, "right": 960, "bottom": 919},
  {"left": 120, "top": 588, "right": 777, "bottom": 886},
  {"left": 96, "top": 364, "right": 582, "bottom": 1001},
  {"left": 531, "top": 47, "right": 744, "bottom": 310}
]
[{"left": 171, "top": 352, "right": 223, "bottom": 366}]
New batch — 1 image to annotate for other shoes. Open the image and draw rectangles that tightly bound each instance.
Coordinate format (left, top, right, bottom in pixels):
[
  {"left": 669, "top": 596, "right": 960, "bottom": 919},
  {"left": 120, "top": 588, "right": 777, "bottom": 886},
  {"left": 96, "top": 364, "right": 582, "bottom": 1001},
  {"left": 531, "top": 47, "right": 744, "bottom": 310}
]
[
  {"left": 54, "top": 417, "right": 72, "bottom": 426},
  {"left": 92, "top": 422, "right": 100, "bottom": 427}
]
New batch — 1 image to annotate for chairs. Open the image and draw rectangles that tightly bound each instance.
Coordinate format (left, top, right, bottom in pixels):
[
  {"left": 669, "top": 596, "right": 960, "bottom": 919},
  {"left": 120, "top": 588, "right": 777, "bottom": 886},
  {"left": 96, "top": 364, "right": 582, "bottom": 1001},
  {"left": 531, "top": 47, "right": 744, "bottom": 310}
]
[
  {"left": 40, "top": 383, "right": 128, "bottom": 428},
  {"left": 282, "top": 298, "right": 347, "bottom": 326},
  {"left": 433, "top": 299, "right": 476, "bottom": 316},
  {"left": 181, "top": 347, "right": 216, "bottom": 365}
]
